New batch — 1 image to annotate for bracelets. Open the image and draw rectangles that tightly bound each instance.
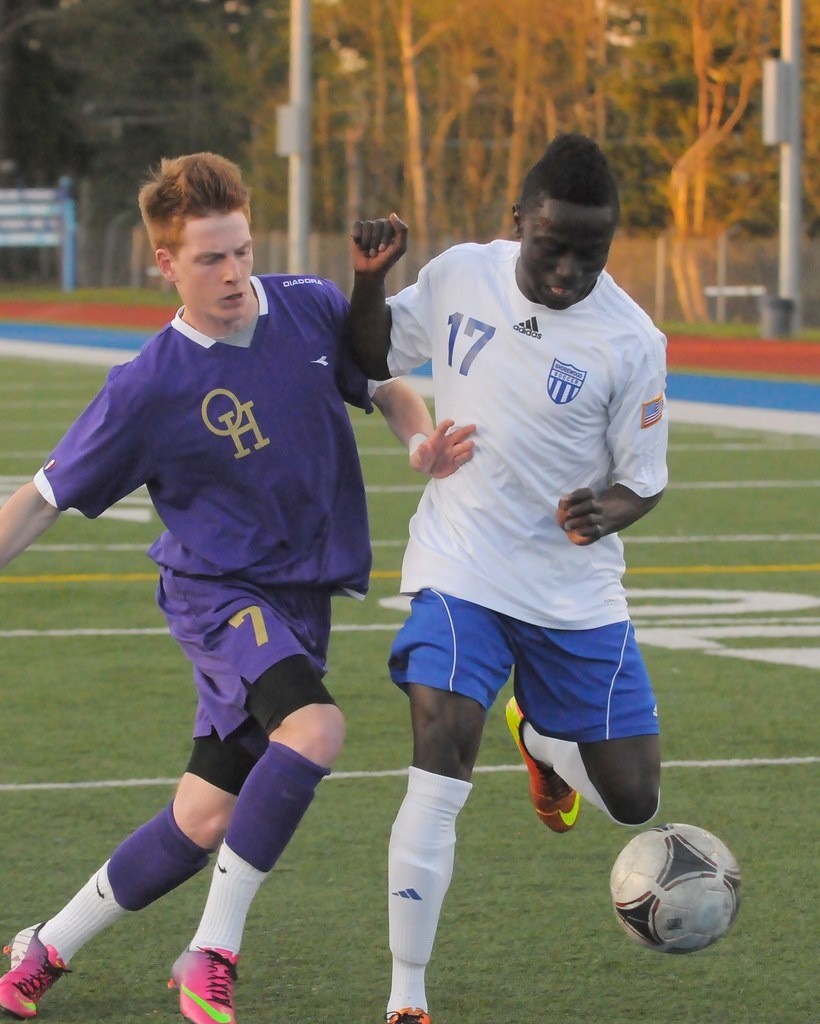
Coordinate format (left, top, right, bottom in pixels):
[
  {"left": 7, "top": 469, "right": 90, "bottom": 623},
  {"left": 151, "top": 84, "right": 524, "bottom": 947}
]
[{"left": 409, "top": 433, "right": 427, "bottom": 455}]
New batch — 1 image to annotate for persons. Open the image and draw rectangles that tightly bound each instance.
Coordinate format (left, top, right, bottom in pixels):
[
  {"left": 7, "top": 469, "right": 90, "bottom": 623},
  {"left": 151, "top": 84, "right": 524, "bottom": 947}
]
[
  {"left": 346, "top": 129, "right": 670, "bottom": 1024},
  {"left": 1, "top": 152, "right": 479, "bottom": 1024}
]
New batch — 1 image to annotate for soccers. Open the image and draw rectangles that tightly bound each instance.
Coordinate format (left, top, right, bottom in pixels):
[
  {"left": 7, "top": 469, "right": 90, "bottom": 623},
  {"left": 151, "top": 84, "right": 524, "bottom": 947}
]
[{"left": 608, "top": 821, "right": 744, "bottom": 956}]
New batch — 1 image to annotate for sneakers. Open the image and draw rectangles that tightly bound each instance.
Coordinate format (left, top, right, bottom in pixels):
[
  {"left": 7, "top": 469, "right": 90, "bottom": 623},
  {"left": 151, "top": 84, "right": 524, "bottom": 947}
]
[
  {"left": 0, "top": 922, "right": 74, "bottom": 1018},
  {"left": 167, "top": 945, "right": 240, "bottom": 1024},
  {"left": 383, "top": 1007, "right": 430, "bottom": 1024},
  {"left": 505, "top": 696, "right": 581, "bottom": 833}
]
